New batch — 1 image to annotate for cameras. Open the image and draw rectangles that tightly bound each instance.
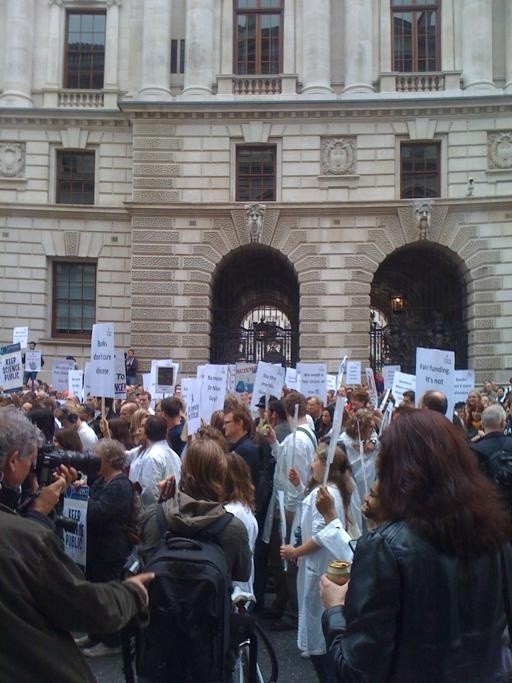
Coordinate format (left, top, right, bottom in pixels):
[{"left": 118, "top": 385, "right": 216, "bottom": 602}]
[
  {"left": 53, "top": 513, "right": 77, "bottom": 534},
  {"left": 37, "top": 441, "right": 102, "bottom": 488}
]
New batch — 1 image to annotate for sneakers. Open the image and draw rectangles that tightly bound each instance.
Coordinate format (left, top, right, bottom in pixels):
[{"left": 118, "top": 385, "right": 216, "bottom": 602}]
[
  {"left": 74, "top": 636, "right": 94, "bottom": 647},
  {"left": 83, "top": 642, "right": 121, "bottom": 657}
]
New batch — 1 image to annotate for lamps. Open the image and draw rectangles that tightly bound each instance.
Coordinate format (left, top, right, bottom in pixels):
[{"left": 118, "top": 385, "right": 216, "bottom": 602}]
[{"left": 390, "top": 290, "right": 407, "bottom": 314}]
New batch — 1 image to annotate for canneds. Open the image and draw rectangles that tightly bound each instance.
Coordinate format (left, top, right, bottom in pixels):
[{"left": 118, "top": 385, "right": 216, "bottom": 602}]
[{"left": 327, "top": 560, "right": 351, "bottom": 588}]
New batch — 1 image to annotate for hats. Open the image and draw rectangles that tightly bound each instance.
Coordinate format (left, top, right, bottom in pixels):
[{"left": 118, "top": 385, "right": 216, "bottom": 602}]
[
  {"left": 24, "top": 408, "right": 54, "bottom": 430},
  {"left": 254, "top": 395, "right": 277, "bottom": 408}
]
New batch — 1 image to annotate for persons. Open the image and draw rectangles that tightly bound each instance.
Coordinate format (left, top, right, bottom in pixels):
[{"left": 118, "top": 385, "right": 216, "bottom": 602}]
[
  {"left": 244, "top": 204, "right": 265, "bottom": 241},
  {"left": 413, "top": 201, "right": 432, "bottom": 237},
  {"left": 22, "top": 342, "right": 44, "bottom": 384},
  {"left": 124, "top": 349, "right": 138, "bottom": 385}
]
